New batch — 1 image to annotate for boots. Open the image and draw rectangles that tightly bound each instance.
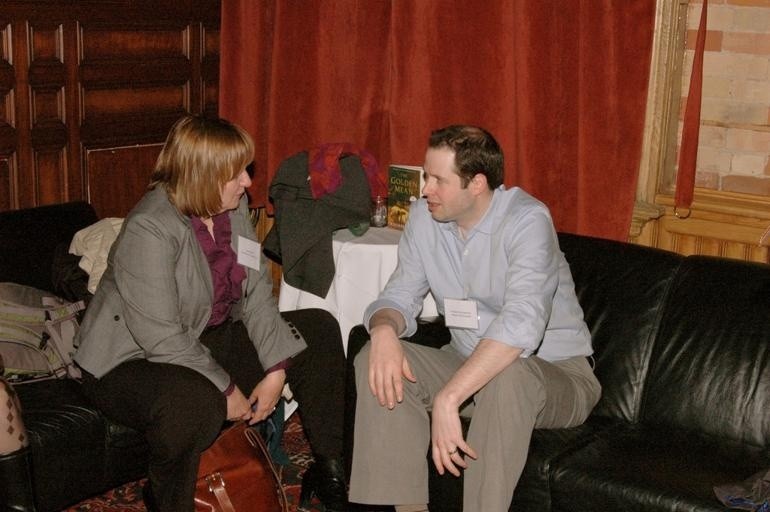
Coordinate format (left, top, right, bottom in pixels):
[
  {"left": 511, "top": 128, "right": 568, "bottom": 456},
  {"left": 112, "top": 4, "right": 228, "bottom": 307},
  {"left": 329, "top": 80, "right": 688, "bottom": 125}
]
[{"left": 0, "top": 442, "right": 41, "bottom": 511}]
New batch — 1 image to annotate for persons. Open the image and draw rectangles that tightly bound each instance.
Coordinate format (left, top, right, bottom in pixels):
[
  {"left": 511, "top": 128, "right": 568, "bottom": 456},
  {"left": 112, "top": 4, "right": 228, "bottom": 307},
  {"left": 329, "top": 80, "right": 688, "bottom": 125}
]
[
  {"left": 347, "top": 125, "right": 606, "bottom": 512},
  {"left": 74, "top": 112, "right": 345, "bottom": 512}
]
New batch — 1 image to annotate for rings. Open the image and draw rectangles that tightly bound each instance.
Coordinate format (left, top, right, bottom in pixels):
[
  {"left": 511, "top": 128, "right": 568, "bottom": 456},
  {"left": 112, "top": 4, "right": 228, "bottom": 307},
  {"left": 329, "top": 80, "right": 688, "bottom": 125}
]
[
  {"left": 448, "top": 447, "right": 458, "bottom": 456},
  {"left": 270, "top": 406, "right": 276, "bottom": 412}
]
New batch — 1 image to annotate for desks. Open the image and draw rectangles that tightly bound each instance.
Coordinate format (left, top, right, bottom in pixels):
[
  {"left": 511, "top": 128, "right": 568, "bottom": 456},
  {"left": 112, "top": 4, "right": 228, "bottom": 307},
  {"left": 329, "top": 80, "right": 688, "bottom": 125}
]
[{"left": 274, "top": 224, "right": 415, "bottom": 327}]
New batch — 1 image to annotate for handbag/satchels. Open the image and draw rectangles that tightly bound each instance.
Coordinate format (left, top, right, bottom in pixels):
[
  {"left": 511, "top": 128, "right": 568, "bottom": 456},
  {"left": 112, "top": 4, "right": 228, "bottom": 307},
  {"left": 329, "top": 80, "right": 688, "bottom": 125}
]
[
  {"left": 194, "top": 421, "right": 289, "bottom": 512},
  {"left": 1, "top": 282, "right": 86, "bottom": 383}
]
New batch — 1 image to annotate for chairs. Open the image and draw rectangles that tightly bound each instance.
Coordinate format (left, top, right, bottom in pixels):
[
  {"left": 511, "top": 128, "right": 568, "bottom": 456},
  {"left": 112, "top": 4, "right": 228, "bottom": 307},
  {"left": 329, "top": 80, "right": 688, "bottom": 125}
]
[{"left": 0, "top": 200, "right": 223, "bottom": 511}]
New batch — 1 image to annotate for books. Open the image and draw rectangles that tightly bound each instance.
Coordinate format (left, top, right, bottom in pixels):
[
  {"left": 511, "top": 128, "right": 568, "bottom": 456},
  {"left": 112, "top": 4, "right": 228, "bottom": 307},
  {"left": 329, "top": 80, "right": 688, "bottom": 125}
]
[{"left": 385, "top": 164, "right": 427, "bottom": 233}]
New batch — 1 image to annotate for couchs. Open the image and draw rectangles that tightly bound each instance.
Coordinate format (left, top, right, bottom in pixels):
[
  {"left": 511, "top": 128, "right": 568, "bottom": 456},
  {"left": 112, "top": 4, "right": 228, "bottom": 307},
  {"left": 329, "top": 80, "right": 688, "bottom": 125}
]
[{"left": 350, "top": 228, "right": 768, "bottom": 510}]
